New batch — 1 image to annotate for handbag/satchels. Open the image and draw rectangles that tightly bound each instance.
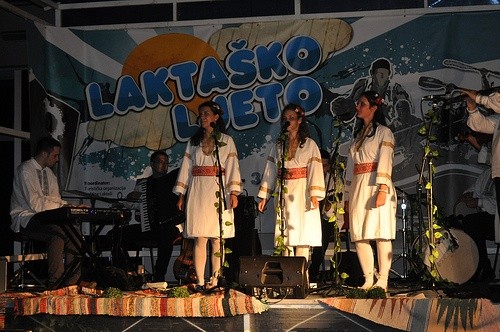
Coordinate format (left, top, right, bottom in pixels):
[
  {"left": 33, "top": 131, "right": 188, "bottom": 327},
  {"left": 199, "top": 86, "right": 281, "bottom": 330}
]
[{"left": 103, "top": 248, "right": 140, "bottom": 289}]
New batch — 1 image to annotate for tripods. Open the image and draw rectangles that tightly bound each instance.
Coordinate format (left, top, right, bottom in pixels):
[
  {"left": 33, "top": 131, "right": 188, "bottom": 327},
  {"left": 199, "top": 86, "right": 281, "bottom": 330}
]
[
  {"left": 387, "top": 191, "right": 433, "bottom": 284},
  {"left": 307, "top": 121, "right": 361, "bottom": 295}
]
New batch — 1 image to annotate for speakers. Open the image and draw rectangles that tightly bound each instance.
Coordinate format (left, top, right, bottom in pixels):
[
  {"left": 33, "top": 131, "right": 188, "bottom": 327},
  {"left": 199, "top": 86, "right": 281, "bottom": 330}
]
[{"left": 238, "top": 254, "right": 307, "bottom": 298}]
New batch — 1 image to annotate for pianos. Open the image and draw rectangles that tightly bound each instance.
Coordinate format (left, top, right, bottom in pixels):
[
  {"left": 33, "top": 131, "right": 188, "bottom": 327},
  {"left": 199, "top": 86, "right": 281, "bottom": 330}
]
[{"left": 46, "top": 206, "right": 133, "bottom": 288}]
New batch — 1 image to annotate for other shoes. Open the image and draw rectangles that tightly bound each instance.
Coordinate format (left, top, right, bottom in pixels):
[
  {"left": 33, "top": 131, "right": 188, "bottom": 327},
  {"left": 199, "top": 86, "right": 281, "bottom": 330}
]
[
  {"left": 187, "top": 282, "right": 218, "bottom": 294},
  {"left": 346, "top": 286, "right": 389, "bottom": 299}
]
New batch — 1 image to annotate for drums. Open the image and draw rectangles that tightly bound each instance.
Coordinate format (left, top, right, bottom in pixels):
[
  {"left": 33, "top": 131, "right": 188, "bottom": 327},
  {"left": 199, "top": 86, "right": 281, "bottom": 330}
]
[{"left": 411, "top": 226, "right": 480, "bottom": 285}]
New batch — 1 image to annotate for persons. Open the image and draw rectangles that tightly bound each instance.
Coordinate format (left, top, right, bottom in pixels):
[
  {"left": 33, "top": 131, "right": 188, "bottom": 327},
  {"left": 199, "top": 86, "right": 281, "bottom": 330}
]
[
  {"left": 106, "top": 151, "right": 188, "bottom": 283},
  {"left": 460, "top": 87, "right": 500, "bottom": 220},
  {"left": 173, "top": 101, "right": 242, "bottom": 288},
  {"left": 257, "top": 102, "right": 326, "bottom": 289},
  {"left": 146, "top": 150, "right": 185, "bottom": 283},
  {"left": 343, "top": 90, "right": 397, "bottom": 299},
  {"left": 9, "top": 136, "right": 97, "bottom": 290}
]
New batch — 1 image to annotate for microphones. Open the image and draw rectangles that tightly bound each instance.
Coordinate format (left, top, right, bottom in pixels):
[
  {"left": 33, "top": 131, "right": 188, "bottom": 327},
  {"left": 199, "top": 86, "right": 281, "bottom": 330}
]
[
  {"left": 334, "top": 111, "right": 357, "bottom": 121},
  {"left": 210, "top": 122, "right": 217, "bottom": 129},
  {"left": 423, "top": 94, "right": 451, "bottom": 100},
  {"left": 280, "top": 121, "right": 290, "bottom": 134}
]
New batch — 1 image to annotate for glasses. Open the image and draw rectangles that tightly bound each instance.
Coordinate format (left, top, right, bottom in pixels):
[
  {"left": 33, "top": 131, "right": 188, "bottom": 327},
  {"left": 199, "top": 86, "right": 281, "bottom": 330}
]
[{"left": 200, "top": 111, "right": 214, "bottom": 117}]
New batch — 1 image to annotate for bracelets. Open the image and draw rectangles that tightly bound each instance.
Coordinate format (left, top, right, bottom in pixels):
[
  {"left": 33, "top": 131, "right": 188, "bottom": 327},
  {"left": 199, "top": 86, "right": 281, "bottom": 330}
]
[
  {"left": 179, "top": 195, "right": 183, "bottom": 198},
  {"left": 378, "top": 189, "right": 387, "bottom": 193},
  {"left": 231, "top": 194, "right": 237, "bottom": 197}
]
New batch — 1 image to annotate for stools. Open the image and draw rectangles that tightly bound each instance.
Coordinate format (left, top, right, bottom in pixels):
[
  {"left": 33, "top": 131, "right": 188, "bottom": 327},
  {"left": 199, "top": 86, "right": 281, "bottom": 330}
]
[
  {"left": 128, "top": 235, "right": 159, "bottom": 283},
  {"left": 4, "top": 230, "right": 48, "bottom": 292}
]
